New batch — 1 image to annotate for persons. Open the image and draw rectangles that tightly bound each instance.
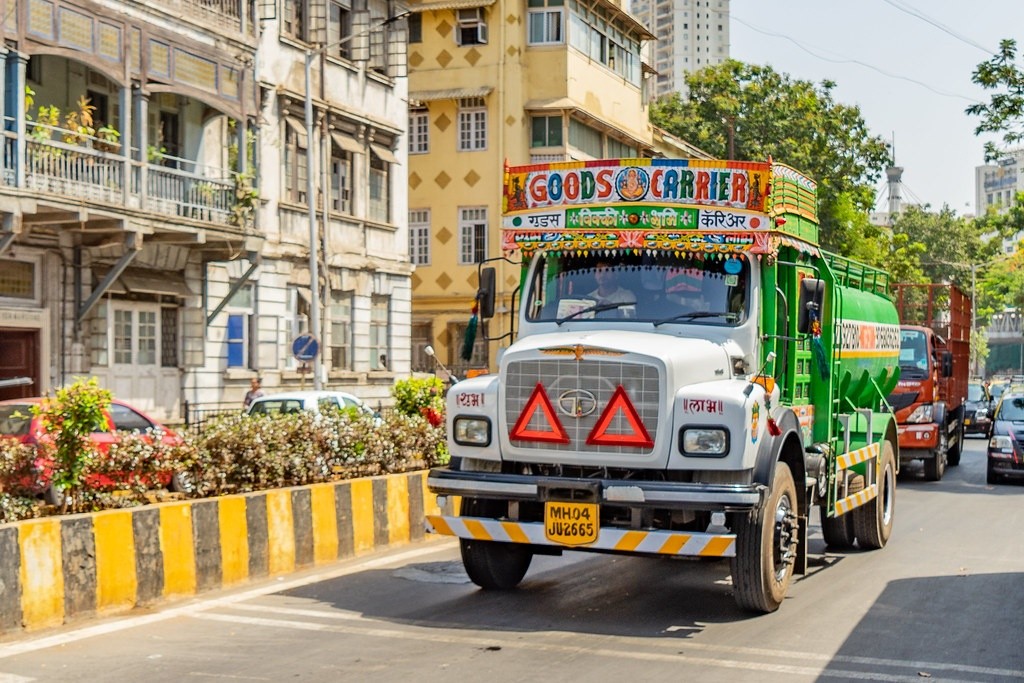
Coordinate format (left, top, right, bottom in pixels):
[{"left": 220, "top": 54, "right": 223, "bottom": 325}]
[
  {"left": 586, "top": 263, "right": 637, "bottom": 317},
  {"left": 243, "top": 378, "right": 265, "bottom": 413}
]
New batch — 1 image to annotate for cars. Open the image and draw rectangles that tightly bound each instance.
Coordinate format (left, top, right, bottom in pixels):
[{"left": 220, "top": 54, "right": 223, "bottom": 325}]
[
  {"left": 0, "top": 395, "right": 206, "bottom": 507},
  {"left": 985, "top": 397, "right": 1024, "bottom": 484},
  {"left": 962, "top": 374, "right": 1024, "bottom": 438},
  {"left": 246, "top": 389, "right": 386, "bottom": 429}
]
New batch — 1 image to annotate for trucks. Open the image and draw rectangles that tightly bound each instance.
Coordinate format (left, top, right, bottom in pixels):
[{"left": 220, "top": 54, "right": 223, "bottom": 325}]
[
  {"left": 422, "top": 225, "right": 903, "bottom": 614},
  {"left": 888, "top": 283, "right": 972, "bottom": 481}
]
